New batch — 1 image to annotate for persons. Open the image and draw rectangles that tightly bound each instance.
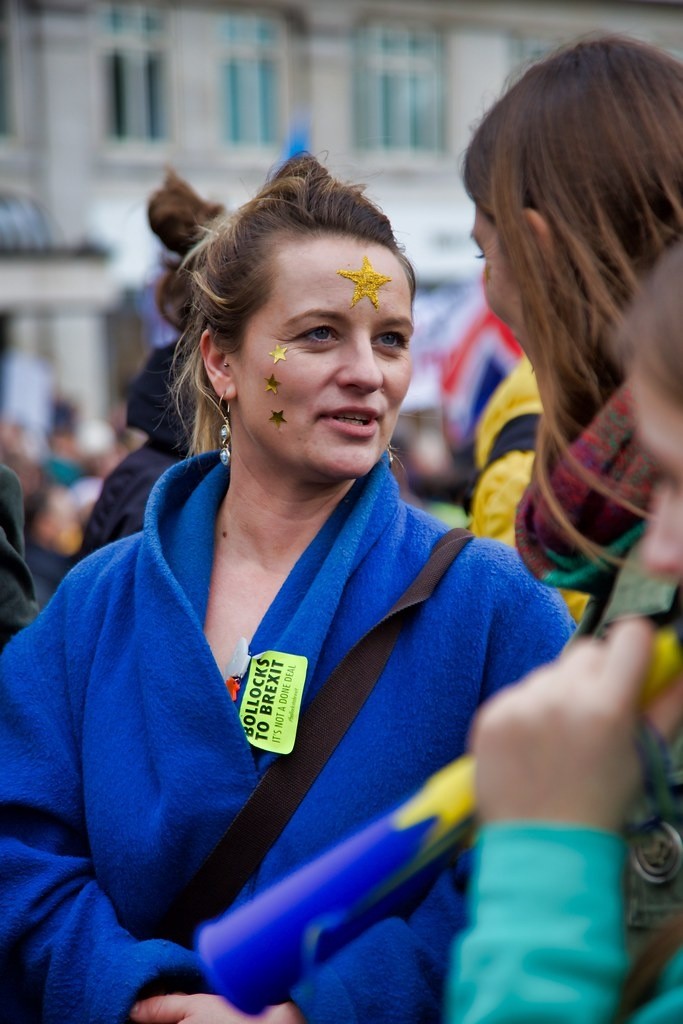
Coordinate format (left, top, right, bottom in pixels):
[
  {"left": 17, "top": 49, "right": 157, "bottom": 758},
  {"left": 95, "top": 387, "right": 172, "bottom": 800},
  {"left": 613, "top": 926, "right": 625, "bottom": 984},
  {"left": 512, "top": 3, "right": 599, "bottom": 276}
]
[
  {"left": 460, "top": 31, "right": 681, "bottom": 930},
  {"left": 440, "top": 235, "right": 683, "bottom": 1024},
  {"left": 0, "top": 150, "right": 577, "bottom": 1023},
  {"left": 0, "top": 29, "right": 683, "bottom": 1024}
]
